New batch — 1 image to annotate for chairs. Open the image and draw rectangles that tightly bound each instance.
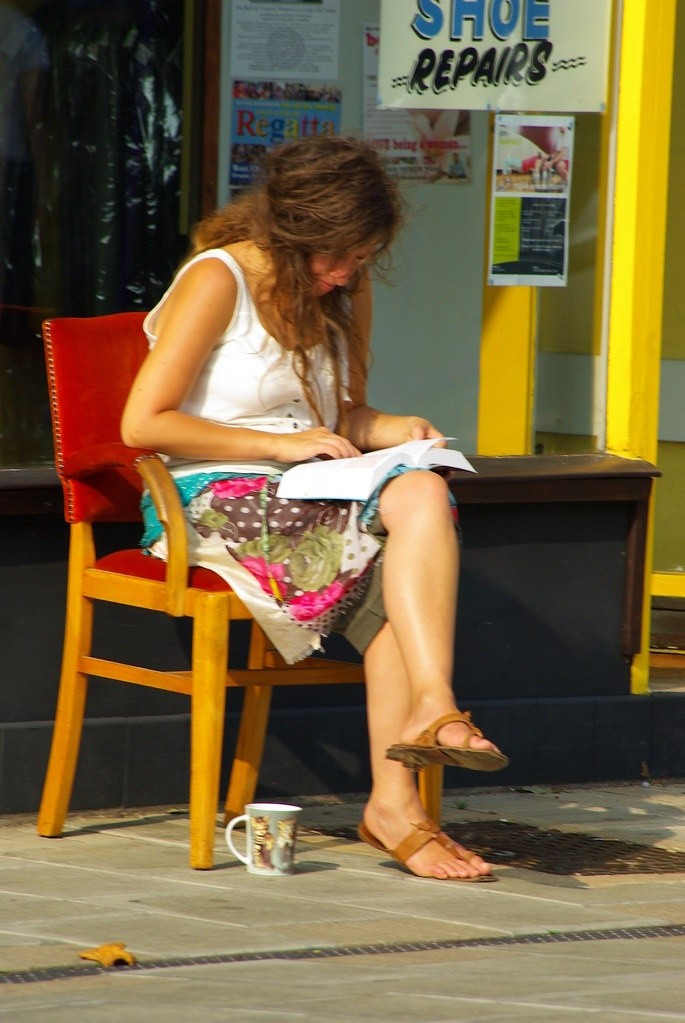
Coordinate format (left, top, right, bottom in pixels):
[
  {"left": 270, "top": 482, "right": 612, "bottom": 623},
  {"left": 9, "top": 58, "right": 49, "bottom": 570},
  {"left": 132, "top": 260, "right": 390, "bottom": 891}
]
[{"left": 38, "top": 311, "right": 444, "bottom": 869}]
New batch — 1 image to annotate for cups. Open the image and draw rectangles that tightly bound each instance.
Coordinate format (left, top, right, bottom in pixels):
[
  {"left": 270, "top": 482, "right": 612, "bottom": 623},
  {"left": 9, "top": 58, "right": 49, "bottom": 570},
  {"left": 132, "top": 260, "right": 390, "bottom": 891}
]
[{"left": 225, "top": 802, "right": 303, "bottom": 877}]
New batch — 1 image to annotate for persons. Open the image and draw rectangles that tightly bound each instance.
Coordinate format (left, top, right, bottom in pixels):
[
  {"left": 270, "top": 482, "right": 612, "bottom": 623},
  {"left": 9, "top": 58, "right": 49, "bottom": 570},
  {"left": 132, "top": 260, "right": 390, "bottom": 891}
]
[
  {"left": 448, "top": 152, "right": 466, "bottom": 178},
  {"left": 1, "top": 0, "right": 55, "bottom": 347},
  {"left": 531, "top": 126, "right": 571, "bottom": 192},
  {"left": 233, "top": 81, "right": 340, "bottom": 103},
  {"left": 121, "top": 134, "right": 510, "bottom": 882},
  {"left": 231, "top": 144, "right": 265, "bottom": 163},
  {"left": 410, "top": 109, "right": 470, "bottom": 182}
]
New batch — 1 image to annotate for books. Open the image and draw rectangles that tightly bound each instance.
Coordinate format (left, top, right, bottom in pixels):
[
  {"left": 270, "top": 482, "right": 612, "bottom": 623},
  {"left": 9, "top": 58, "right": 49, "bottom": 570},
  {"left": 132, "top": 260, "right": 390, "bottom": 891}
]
[{"left": 275, "top": 437, "right": 478, "bottom": 502}]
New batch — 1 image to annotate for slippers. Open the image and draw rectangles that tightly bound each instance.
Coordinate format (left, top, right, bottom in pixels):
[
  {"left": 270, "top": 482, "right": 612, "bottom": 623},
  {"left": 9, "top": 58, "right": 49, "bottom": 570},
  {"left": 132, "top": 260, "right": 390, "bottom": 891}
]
[
  {"left": 385, "top": 710, "right": 510, "bottom": 772},
  {"left": 357, "top": 818, "right": 499, "bottom": 883}
]
